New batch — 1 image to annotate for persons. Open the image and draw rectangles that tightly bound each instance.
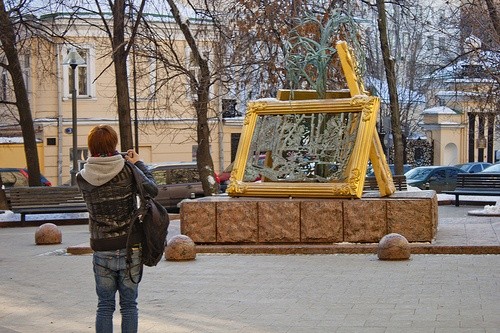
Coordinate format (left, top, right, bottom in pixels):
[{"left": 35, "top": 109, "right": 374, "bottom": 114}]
[{"left": 76, "top": 123, "right": 158, "bottom": 333}]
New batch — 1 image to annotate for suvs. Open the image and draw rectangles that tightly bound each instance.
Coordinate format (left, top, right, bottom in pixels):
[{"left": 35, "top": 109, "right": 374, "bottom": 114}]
[
  {"left": 149, "top": 162, "right": 204, "bottom": 210},
  {"left": 219, "top": 154, "right": 265, "bottom": 193}
]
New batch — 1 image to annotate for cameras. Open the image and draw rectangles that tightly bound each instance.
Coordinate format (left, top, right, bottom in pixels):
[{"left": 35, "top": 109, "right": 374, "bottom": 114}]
[{"left": 118, "top": 152, "right": 129, "bottom": 161}]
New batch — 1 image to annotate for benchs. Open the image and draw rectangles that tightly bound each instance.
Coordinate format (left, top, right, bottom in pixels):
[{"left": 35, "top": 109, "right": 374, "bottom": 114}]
[
  {"left": 2, "top": 186, "right": 88, "bottom": 223},
  {"left": 441, "top": 173, "right": 500, "bottom": 206},
  {"left": 363, "top": 175, "right": 408, "bottom": 191}
]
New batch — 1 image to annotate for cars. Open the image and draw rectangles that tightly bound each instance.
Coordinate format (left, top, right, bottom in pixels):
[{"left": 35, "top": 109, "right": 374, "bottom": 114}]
[
  {"left": 482, "top": 161, "right": 500, "bottom": 174},
  {"left": 0, "top": 167, "right": 52, "bottom": 187},
  {"left": 368, "top": 164, "right": 413, "bottom": 176},
  {"left": 404, "top": 165, "right": 469, "bottom": 193},
  {"left": 454, "top": 162, "right": 491, "bottom": 174}
]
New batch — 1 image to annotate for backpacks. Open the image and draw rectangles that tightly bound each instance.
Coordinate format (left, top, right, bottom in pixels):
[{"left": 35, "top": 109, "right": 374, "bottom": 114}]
[{"left": 127, "top": 163, "right": 170, "bottom": 284}]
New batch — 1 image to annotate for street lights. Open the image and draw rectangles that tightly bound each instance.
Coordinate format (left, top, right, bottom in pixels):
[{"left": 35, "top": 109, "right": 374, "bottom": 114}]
[
  {"left": 463, "top": 35, "right": 500, "bottom": 56},
  {"left": 60, "top": 46, "right": 87, "bottom": 185}
]
